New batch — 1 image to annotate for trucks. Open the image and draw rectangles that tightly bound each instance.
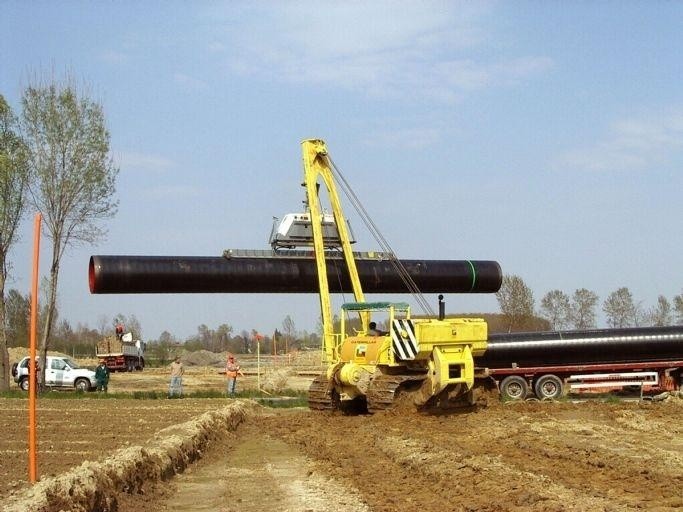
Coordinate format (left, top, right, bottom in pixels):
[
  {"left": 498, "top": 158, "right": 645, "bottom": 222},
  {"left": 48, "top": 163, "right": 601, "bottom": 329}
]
[{"left": 95, "top": 339, "right": 146, "bottom": 372}]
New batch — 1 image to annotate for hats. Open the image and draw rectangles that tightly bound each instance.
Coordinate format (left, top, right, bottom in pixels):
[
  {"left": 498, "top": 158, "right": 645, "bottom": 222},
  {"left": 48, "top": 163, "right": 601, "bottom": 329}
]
[
  {"left": 229, "top": 355, "right": 234, "bottom": 359},
  {"left": 97, "top": 359, "right": 107, "bottom": 363}
]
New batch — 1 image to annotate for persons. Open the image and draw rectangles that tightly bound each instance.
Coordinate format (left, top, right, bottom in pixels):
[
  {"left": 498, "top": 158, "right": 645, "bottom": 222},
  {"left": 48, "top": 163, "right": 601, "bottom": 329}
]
[
  {"left": 94, "top": 359, "right": 109, "bottom": 393},
  {"left": 368, "top": 321, "right": 381, "bottom": 336},
  {"left": 116, "top": 324, "right": 123, "bottom": 339},
  {"left": 226, "top": 355, "right": 240, "bottom": 392},
  {"left": 168, "top": 354, "right": 185, "bottom": 396}
]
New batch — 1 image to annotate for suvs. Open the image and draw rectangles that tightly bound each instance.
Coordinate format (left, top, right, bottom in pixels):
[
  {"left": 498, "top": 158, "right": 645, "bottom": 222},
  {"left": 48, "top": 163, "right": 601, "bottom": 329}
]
[{"left": 12, "top": 356, "right": 98, "bottom": 392}]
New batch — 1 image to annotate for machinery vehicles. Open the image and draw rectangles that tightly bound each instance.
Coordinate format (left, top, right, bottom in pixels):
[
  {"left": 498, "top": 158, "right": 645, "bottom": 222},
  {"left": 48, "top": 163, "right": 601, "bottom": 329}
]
[{"left": 271, "top": 139, "right": 499, "bottom": 415}]
